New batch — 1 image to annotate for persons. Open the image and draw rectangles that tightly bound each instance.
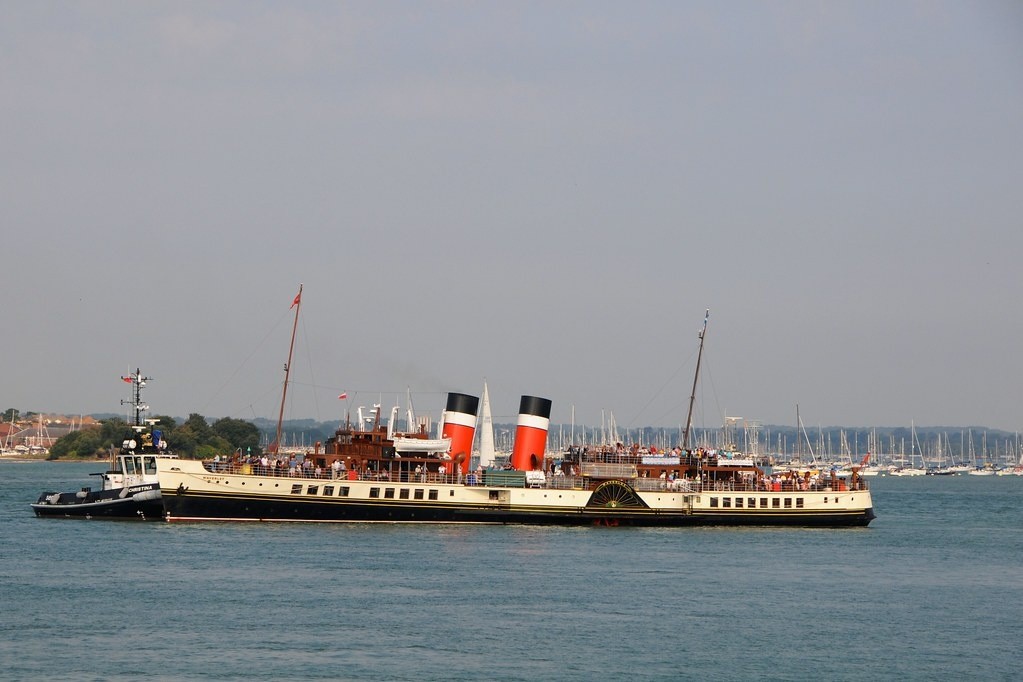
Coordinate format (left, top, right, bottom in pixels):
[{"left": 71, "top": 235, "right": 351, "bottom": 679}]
[
  {"left": 212, "top": 450, "right": 389, "bottom": 481},
  {"left": 438, "top": 465, "right": 446, "bottom": 483},
  {"left": 547, "top": 461, "right": 576, "bottom": 478},
  {"left": 474, "top": 463, "right": 517, "bottom": 485},
  {"left": 457, "top": 465, "right": 463, "bottom": 484},
  {"left": 569, "top": 442, "right": 752, "bottom": 465},
  {"left": 659, "top": 469, "right": 820, "bottom": 491},
  {"left": 415, "top": 464, "right": 428, "bottom": 483}
]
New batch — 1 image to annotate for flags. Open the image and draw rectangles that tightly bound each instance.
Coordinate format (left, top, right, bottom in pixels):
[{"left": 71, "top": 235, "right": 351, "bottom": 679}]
[
  {"left": 338, "top": 393, "right": 346, "bottom": 400},
  {"left": 290, "top": 294, "right": 300, "bottom": 309}
]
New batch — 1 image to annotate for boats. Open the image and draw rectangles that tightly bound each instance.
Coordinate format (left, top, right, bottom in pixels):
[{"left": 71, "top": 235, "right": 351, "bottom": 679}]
[
  {"left": 26, "top": 484, "right": 162, "bottom": 520},
  {"left": 100, "top": 371, "right": 178, "bottom": 492},
  {"left": 154, "top": 282, "right": 881, "bottom": 527},
  {"left": 767, "top": 404, "right": 1023, "bottom": 477}
]
